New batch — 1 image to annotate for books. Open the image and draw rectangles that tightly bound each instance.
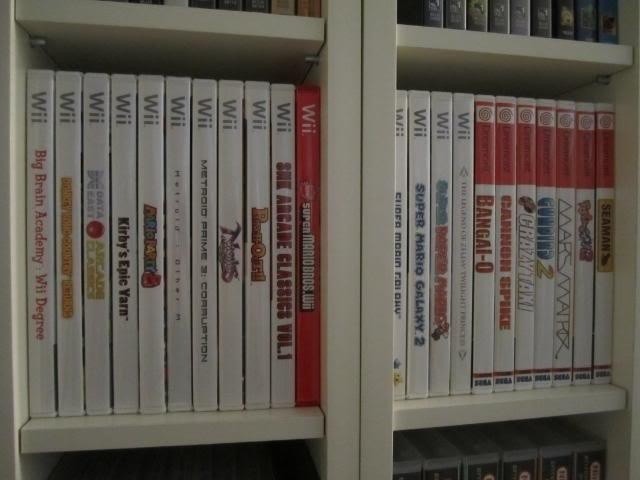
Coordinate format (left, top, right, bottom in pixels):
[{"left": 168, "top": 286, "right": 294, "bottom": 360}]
[
  {"left": 390, "top": 418, "right": 608, "bottom": 479},
  {"left": 188, "top": 1, "right": 323, "bottom": 20},
  {"left": 412, "top": 0, "right": 619, "bottom": 45}
]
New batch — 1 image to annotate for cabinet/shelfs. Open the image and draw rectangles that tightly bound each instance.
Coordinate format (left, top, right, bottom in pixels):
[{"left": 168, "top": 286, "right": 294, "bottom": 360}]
[{"left": 1, "top": 0, "right": 639, "bottom": 480}]
